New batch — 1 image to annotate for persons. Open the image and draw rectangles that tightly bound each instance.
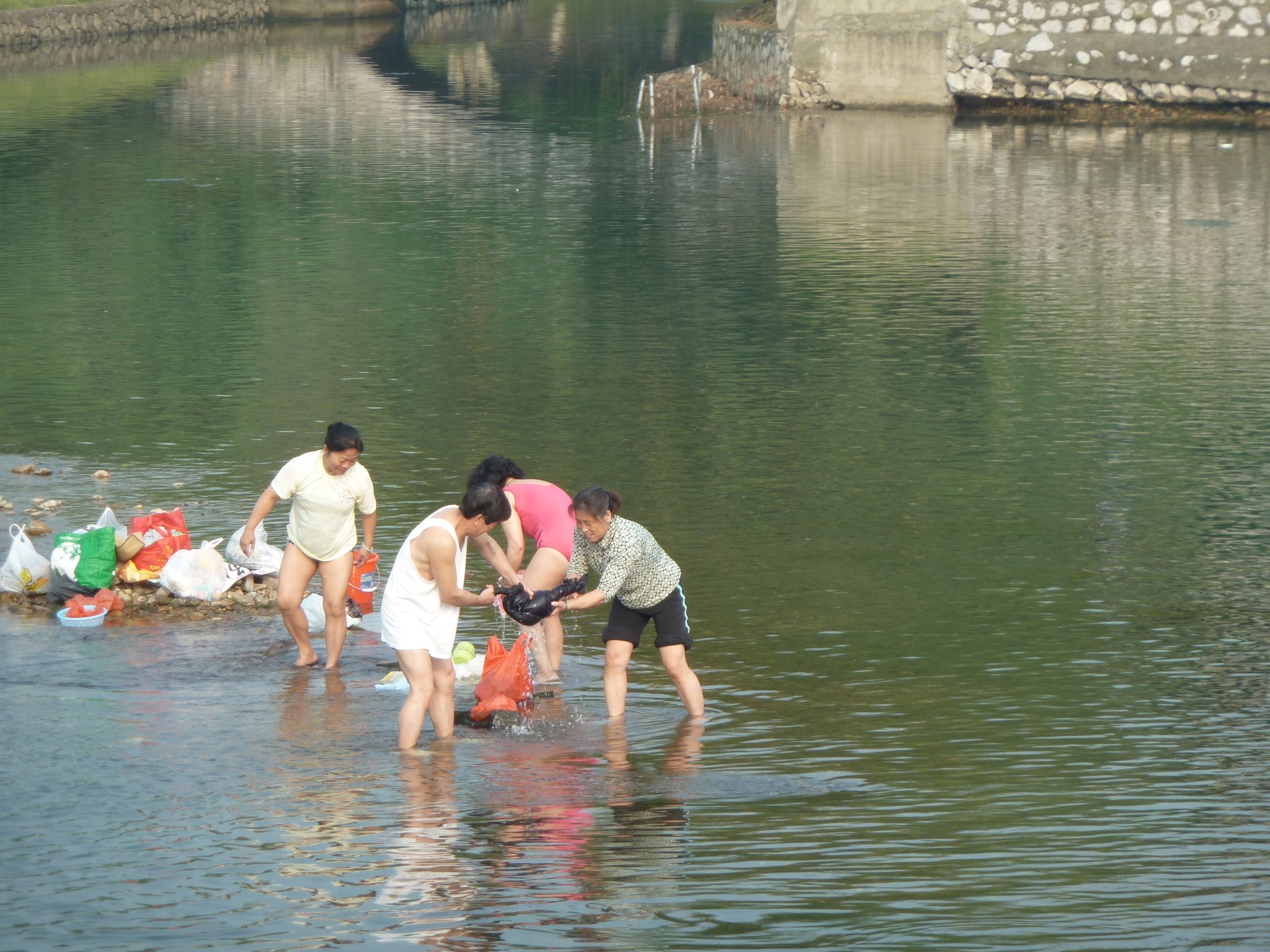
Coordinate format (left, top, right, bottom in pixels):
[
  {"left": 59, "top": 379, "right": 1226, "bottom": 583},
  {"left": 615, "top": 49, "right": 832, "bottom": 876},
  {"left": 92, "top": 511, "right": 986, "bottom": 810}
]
[
  {"left": 239, "top": 421, "right": 377, "bottom": 668},
  {"left": 467, "top": 454, "right": 577, "bottom": 685},
  {"left": 550, "top": 488, "right": 703, "bottom": 716},
  {"left": 380, "top": 481, "right": 534, "bottom": 750}
]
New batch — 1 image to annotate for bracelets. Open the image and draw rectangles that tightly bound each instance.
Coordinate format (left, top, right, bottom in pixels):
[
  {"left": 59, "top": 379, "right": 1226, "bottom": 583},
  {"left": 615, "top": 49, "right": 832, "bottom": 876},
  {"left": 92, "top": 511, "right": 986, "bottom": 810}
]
[
  {"left": 362, "top": 542, "right": 373, "bottom": 552},
  {"left": 501, "top": 577, "right": 511, "bottom": 588},
  {"left": 559, "top": 599, "right": 568, "bottom": 614}
]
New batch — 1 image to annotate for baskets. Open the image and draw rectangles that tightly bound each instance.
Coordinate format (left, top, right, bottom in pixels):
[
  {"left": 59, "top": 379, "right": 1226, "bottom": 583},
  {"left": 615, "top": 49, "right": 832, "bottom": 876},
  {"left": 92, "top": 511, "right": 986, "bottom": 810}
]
[{"left": 56, "top": 606, "right": 107, "bottom": 628}]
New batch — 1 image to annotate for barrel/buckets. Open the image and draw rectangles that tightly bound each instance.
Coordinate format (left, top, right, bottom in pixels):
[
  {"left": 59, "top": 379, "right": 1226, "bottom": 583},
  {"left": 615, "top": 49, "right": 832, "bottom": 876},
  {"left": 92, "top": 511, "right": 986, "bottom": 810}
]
[{"left": 347, "top": 550, "right": 380, "bottom": 604}]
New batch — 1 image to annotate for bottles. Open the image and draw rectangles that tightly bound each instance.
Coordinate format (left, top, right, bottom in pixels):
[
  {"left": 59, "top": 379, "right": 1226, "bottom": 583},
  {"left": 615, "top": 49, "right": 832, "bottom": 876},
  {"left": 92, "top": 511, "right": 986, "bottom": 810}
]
[{"left": 453, "top": 641, "right": 476, "bottom": 664}]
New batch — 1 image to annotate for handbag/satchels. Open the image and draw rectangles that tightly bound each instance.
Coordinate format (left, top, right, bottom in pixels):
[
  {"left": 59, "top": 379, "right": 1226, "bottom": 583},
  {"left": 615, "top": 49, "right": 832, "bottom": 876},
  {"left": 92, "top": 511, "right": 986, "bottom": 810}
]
[
  {"left": 161, "top": 536, "right": 226, "bottom": 601},
  {"left": 299, "top": 594, "right": 361, "bottom": 633},
  {"left": 474, "top": 632, "right": 533, "bottom": 702},
  {"left": 46, "top": 507, "right": 191, "bottom": 611},
  {"left": 0, "top": 523, "right": 52, "bottom": 596},
  {"left": 226, "top": 516, "right": 285, "bottom": 577}
]
